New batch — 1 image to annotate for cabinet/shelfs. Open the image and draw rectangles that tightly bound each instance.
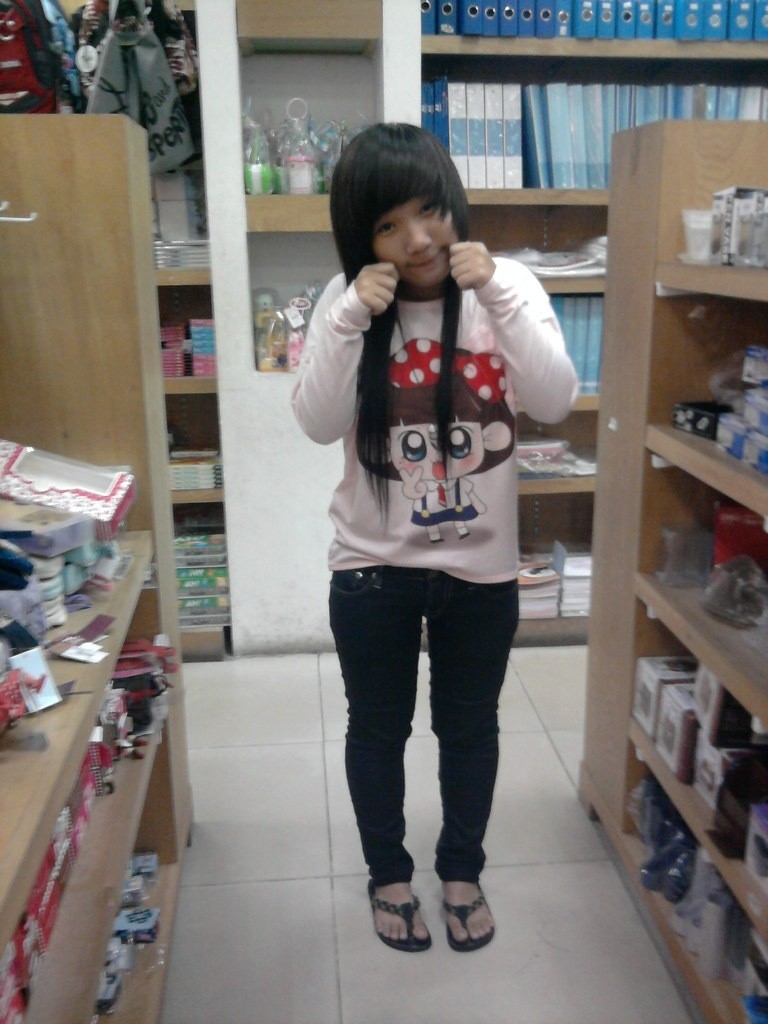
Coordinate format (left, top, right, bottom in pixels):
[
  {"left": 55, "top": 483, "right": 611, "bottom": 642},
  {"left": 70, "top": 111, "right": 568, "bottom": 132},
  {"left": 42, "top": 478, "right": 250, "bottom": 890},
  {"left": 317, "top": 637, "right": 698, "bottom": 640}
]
[{"left": 1, "top": 0, "right": 768, "bottom": 1024}]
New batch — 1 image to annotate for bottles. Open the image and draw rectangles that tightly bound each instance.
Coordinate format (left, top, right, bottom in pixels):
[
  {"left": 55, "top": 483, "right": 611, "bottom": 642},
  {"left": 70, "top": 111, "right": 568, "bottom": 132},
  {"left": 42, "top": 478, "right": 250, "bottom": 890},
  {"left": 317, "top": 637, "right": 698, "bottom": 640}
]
[{"left": 252, "top": 287, "right": 289, "bottom": 372}]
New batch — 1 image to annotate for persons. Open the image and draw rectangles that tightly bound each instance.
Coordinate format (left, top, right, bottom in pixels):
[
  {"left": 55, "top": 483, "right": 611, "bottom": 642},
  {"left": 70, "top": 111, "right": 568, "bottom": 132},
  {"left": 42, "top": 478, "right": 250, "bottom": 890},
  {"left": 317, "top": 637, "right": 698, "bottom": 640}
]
[{"left": 290, "top": 121, "right": 577, "bottom": 955}]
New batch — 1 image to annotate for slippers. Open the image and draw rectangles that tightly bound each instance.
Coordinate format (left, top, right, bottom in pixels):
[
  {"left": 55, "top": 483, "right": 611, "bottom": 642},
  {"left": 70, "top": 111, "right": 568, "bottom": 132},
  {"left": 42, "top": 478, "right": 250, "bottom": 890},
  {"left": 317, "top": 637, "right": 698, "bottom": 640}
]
[
  {"left": 368, "top": 877, "right": 432, "bottom": 953},
  {"left": 441, "top": 874, "right": 495, "bottom": 953}
]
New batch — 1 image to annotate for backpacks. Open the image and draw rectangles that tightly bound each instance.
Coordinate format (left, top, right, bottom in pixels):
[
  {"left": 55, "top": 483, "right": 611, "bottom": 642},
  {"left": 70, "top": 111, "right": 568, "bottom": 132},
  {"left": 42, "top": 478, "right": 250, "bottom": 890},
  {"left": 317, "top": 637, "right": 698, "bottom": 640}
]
[{"left": 0, "top": 0, "right": 80, "bottom": 115}]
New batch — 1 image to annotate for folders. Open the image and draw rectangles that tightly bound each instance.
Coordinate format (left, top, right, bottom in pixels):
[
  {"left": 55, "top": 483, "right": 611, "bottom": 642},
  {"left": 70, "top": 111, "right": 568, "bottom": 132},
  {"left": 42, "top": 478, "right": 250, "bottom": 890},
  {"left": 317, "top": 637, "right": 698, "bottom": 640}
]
[
  {"left": 444, "top": 81, "right": 768, "bottom": 190},
  {"left": 421, "top": 0, "right": 768, "bottom": 43}
]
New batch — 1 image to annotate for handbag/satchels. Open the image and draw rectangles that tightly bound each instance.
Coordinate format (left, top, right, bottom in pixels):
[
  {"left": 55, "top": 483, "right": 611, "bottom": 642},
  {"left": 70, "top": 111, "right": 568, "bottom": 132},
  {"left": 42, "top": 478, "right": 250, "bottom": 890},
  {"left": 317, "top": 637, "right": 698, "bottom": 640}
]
[{"left": 76, "top": 0, "right": 202, "bottom": 175}]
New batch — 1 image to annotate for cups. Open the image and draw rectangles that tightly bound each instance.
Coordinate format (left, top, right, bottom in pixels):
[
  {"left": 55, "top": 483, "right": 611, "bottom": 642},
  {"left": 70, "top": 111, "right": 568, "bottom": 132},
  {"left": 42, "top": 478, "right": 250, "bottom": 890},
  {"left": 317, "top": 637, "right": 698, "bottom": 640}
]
[
  {"left": 735, "top": 213, "right": 768, "bottom": 269},
  {"left": 681, "top": 207, "right": 717, "bottom": 262}
]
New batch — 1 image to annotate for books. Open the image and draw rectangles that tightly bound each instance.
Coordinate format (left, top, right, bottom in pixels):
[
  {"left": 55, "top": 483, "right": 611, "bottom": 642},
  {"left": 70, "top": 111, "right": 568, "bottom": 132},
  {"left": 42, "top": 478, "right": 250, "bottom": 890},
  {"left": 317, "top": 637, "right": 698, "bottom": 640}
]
[{"left": 518, "top": 556, "right": 592, "bottom": 619}]
